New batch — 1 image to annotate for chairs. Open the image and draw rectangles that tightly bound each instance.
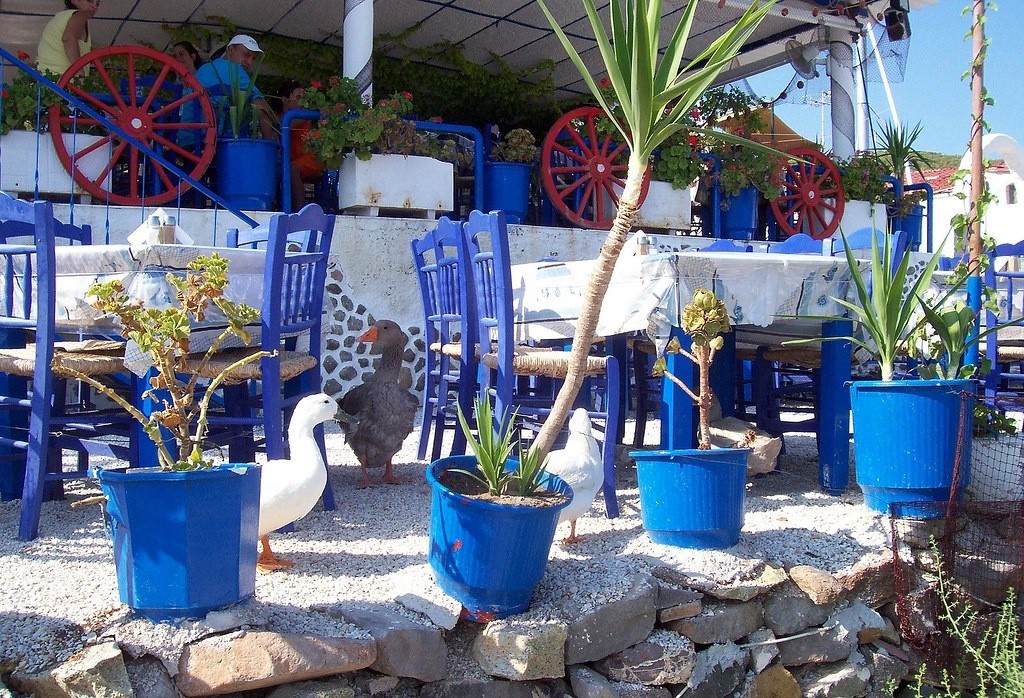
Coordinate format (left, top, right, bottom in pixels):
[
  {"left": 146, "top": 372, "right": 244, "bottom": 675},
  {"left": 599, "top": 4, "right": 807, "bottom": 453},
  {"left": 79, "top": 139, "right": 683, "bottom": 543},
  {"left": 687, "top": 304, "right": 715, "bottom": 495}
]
[
  {"left": 118, "top": 75, "right": 184, "bottom": 207},
  {"left": 192, "top": 83, "right": 254, "bottom": 209},
  {"left": 0, "top": 190, "right": 1024, "bottom": 541},
  {"left": 542, "top": 129, "right": 583, "bottom": 227}
]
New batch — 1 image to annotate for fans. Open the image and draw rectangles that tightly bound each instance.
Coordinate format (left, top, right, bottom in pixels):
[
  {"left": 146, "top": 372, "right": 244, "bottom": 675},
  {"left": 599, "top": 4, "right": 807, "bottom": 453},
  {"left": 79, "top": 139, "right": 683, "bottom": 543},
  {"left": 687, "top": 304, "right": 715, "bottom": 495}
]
[{"left": 785, "top": 39, "right": 822, "bottom": 80}]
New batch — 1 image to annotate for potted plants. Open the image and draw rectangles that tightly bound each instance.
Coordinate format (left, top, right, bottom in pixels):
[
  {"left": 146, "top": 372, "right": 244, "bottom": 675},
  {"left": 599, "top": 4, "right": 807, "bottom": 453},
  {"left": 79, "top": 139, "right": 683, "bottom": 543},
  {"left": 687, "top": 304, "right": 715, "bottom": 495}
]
[
  {"left": 963, "top": 403, "right": 1024, "bottom": 502},
  {"left": 47, "top": 251, "right": 280, "bottom": 622},
  {"left": 484, "top": 128, "right": 541, "bottom": 224},
  {"left": 770, "top": 204, "right": 1024, "bottom": 518},
  {"left": 424, "top": 0, "right": 781, "bottom": 623},
  {"left": 870, "top": 116, "right": 936, "bottom": 252},
  {"left": 629, "top": 285, "right": 753, "bottom": 550},
  {"left": 204, "top": 40, "right": 286, "bottom": 212}
]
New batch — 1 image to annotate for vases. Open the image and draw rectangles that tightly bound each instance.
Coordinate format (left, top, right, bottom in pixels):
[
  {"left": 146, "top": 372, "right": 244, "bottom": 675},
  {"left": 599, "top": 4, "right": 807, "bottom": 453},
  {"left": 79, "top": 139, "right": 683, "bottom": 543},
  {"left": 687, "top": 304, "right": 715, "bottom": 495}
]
[
  {"left": 801, "top": 197, "right": 888, "bottom": 239},
  {"left": 0, "top": 130, "right": 113, "bottom": 205},
  {"left": 702, "top": 186, "right": 759, "bottom": 239},
  {"left": 592, "top": 178, "right": 692, "bottom": 235},
  {"left": 338, "top": 152, "right": 455, "bottom": 220}
]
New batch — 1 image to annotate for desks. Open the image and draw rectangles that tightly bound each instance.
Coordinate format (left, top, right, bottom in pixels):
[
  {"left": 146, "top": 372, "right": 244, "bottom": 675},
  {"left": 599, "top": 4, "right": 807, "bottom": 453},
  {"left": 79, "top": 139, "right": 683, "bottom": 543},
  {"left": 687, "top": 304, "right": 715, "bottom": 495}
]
[
  {"left": 0, "top": 242, "right": 336, "bottom": 468},
  {"left": 415, "top": 131, "right": 475, "bottom": 173},
  {"left": 487, "top": 251, "right": 874, "bottom": 497},
  {"left": 932, "top": 271, "right": 1024, "bottom": 324}
]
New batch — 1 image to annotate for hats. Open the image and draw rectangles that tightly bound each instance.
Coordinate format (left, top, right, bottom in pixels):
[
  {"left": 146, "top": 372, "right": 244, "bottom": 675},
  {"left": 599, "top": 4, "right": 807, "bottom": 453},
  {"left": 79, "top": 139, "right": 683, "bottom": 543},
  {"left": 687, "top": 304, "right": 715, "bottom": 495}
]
[{"left": 227, "top": 35, "right": 265, "bottom": 58}]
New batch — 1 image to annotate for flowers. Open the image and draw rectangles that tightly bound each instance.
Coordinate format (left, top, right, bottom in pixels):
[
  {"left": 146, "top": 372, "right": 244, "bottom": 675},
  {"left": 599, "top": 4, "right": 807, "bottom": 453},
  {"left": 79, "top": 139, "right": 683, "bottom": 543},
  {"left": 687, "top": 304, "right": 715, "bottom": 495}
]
[
  {"left": 578, "top": 76, "right": 719, "bottom": 191},
  {"left": 812, "top": 149, "right": 928, "bottom": 218},
  {"left": 710, "top": 126, "right": 787, "bottom": 211},
  {"left": 0, "top": 48, "right": 106, "bottom": 137},
  {"left": 296, "top": 75, "right": 466, "bottom": 172}
]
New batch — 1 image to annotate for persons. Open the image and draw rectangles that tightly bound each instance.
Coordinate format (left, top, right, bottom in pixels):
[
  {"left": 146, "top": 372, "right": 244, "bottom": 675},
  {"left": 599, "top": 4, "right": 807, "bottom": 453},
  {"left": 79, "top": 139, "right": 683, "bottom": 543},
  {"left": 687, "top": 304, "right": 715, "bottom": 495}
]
[
  {"left": 172, "top": 35, "right": 326, "bottom": 214},
  {"left": 34, "top": 0, "right": 103, "bottom": 118}
]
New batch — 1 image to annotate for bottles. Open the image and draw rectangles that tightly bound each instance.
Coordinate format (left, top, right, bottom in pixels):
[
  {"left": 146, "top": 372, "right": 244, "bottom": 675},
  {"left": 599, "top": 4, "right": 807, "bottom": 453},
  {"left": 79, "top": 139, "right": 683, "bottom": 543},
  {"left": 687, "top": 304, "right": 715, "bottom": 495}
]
[
  {"left": 163, "top": 217, "right": 178, "bottom": 244},
  {"left": 146, "top": 215, "right": 164, "bottom": 245}
]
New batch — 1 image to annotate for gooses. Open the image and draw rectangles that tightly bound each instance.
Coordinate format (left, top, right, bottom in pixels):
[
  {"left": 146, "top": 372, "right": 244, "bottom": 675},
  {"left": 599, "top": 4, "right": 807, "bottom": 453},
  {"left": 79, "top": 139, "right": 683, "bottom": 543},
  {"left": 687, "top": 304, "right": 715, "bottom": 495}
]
[
  {"left": 337, "top": 320, "right": 422, "bottom": 490},
  {"left": 247, "top": 393, "right": 362, "bottom": 574},
  {"left": 539, "top": 407, "right": 605, "bottom": 546},
  {"left": 690, "top": 387, "right": 783, "bottom": 479}
]
